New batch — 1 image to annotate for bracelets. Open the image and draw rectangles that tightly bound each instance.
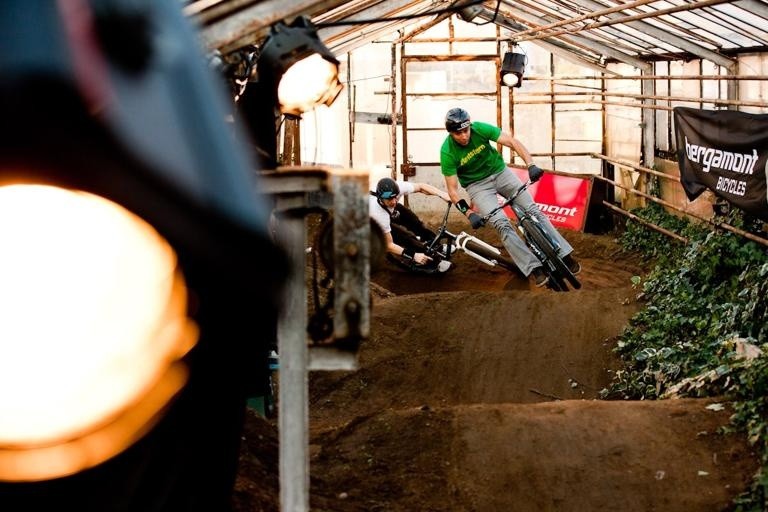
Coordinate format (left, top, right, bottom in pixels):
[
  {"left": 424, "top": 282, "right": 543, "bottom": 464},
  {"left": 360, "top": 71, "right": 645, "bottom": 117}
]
[
  {"left": 401, "top": 248, "right": 416, "bottom": 260},
  {"left": 456, "top": 199, "right": 471, "bottom": 216}
]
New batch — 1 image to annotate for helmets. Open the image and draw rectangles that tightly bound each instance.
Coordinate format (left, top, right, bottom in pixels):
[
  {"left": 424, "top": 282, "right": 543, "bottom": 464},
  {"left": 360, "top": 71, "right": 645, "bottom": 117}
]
[
  {"left": 377, "top": 177, "right": 400, "bottom": 199},
  {"left": 445, "top": 108, "right": 470, "bottom": 133}
]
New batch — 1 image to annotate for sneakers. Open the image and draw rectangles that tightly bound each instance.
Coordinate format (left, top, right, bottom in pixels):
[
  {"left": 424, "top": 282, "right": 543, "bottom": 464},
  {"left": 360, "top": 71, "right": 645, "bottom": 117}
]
[
  {"left": 534, "top": 271, "right": 549, "bottom": 288},
  {"left": 567, "top": 259, "right": 581, "bottom": 275},
  {"left": 442, "top": 243, "right": 456, "bottom": 254},
  {"left": 438, "top": 260, "right": 452, "bottom": 273}
]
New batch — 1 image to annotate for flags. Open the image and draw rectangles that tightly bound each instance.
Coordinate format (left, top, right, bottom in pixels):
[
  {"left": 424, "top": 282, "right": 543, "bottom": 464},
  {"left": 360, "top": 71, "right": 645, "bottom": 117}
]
[
  {"left": 672, "top": 106, "right": 768, "bottom": 203},
  {"left": 472, "top": 167, "right": 591, "bottom": 233}
]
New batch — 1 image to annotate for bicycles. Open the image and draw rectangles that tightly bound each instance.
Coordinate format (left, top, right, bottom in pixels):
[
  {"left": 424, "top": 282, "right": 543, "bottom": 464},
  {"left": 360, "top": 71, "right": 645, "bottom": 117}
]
[
  {"left": 386, "top": 201, "right": 528, "bottom": 281},
  {"left": 473, "top": 170, "right": 582, "bottom": 292}
]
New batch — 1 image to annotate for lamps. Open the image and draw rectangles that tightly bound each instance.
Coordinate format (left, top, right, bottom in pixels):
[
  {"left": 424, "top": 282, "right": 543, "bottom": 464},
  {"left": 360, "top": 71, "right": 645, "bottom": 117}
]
[
  {"left": 447, "top": 1, "right": 484, "bottom": 23},
  {"left": 0, "top": 2, "right": 295, "bottom": 511},
  {"left": 499, "top": 52, "right": 526, "bottom": 89},
  {"left": 257, "top": 16, "right": 341, "bottom": 114}
]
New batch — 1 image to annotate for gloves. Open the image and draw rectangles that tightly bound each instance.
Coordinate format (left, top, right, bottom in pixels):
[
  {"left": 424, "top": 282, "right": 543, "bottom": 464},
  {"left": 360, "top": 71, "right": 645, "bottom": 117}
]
[
  {"left": 528, "top": 164, "right": 544, "bottom": 182},
  {"left": 468, "top": 212, "right": 486, "bottom": 229}
]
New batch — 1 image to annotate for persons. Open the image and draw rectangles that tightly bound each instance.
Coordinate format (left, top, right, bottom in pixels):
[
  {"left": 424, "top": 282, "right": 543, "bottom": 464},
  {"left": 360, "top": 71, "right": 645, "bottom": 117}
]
[
  {"left": 440, "top": 108, "right": 582, "bottom": 287},
  {"left": 365, "top": 177, "right": 456, "bottom": 274}
]
